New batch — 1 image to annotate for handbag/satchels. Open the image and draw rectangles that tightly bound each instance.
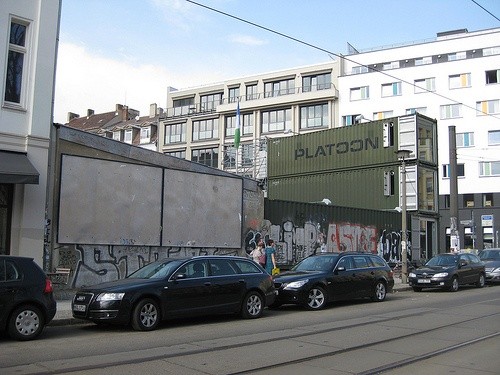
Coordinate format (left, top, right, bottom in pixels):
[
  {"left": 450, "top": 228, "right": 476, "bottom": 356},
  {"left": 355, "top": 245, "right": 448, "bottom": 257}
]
[{"left": 272, "top": 268, "right": 280, "bottom": 275}]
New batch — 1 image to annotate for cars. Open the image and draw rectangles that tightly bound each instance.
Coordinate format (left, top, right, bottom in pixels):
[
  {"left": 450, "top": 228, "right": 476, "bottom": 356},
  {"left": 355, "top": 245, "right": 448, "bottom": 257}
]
[
  {"left": 71, "top": 256, "right": 277, "bottom": 332},
  {"left": 478, "top": 248, "right": 500, "bottom": 284},
  {"left": 408, "top": 253, "right": 486, "bottom": 292},
  {"left": 0, "top": 255, "right": 57, "bottom": 342}
]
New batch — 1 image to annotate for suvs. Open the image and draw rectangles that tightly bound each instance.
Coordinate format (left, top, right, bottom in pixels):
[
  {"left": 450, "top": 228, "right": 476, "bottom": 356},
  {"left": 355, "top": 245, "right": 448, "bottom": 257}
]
[{"left": 267, "top": 251, "right": 394, "bottom": 310}]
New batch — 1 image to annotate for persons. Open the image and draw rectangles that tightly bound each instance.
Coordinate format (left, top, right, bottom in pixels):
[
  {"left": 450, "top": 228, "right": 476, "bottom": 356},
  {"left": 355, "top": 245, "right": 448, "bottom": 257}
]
[
  {"left": 250, "top": 241, "right": 266, "bottom": 268},
  {"left": 264, "top": 239, "right": 276, "bottom": 276}
]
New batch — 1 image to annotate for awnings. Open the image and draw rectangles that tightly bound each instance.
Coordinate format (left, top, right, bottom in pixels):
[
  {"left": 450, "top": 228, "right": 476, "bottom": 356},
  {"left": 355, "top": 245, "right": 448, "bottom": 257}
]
[{"left": 0, "top": 149, "right": 40, "bottom": 185}]
